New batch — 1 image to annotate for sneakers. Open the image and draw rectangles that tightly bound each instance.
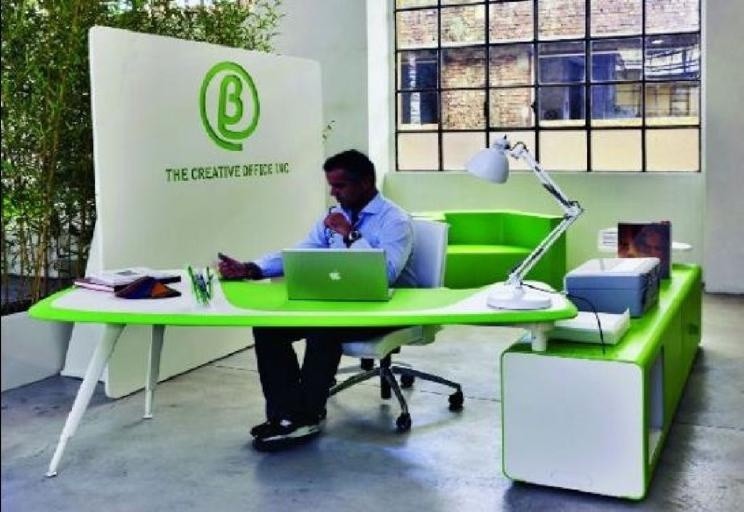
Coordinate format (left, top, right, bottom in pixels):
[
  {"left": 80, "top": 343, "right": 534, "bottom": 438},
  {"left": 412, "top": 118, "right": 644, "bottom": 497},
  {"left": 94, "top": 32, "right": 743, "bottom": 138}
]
[
  {"left": 251, "top": 418, "right": 275, "bottom": 438},
  {"left": 251, "top": 416, "right": 320, "bottom": 452}
]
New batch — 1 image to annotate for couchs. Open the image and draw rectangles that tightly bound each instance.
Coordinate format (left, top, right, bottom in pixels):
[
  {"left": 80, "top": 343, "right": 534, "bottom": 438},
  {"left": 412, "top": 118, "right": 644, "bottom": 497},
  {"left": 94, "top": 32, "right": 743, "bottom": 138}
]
[{"left": 433, "top": 210, "right": 567, "bottom": 291}]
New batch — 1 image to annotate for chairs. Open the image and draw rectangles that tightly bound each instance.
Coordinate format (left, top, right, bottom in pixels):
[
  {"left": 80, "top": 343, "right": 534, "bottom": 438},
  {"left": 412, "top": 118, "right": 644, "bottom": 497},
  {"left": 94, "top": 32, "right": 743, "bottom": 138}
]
[{"left": 330, "top": 220, "right": 465, "bottom": 429}]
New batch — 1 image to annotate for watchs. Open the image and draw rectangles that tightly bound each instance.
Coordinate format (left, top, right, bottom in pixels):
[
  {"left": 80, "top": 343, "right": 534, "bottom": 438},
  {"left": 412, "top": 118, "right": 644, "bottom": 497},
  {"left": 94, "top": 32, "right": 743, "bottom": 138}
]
[{"left": 340, "top": 228, "right": 360, "bottom": 247}]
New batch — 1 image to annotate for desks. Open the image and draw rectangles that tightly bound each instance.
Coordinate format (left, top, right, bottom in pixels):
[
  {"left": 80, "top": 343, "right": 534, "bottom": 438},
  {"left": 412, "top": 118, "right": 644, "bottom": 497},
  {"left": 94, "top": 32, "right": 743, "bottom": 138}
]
[{"left": 27, "top": 269, "right": 577, "bottom": 478}]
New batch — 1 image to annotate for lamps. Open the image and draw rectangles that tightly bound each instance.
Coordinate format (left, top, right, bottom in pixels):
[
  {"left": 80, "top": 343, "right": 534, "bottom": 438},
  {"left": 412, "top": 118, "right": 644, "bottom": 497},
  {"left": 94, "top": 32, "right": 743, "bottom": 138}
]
[{"left": 465, "top": 134, "right": 584, "bottom": 311}]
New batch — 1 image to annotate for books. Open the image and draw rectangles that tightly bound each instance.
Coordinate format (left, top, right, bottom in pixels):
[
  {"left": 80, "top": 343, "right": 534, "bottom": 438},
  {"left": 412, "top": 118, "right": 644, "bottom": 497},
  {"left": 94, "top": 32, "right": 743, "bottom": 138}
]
[{"left": 73, "top": 261, "right": 183, "bottom": 294}]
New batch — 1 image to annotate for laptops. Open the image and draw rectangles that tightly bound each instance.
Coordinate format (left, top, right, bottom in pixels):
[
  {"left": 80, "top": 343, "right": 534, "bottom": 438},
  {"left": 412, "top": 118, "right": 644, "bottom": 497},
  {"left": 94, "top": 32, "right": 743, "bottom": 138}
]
[{"left": 281, "top": 247, "right": 395, "bottom": 301}]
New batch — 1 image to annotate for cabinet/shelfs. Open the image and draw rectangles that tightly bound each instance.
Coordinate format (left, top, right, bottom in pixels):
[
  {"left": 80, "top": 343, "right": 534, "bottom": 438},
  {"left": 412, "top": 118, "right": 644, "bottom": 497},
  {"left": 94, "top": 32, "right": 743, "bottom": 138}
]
[{"left": 501, "top": 262, "right": 701, "bottom": 500}]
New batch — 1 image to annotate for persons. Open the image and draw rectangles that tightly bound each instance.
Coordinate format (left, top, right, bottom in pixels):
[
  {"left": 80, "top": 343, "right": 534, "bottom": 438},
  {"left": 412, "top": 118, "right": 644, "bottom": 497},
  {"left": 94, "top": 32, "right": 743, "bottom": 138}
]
[{"left": 217, "top": 149, "right": 419, "bottom": 452}]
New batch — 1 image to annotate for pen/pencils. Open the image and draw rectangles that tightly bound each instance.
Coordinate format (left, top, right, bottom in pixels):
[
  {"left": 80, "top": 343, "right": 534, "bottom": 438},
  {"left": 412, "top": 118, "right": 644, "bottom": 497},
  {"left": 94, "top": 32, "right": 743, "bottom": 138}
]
[{"left": 188, "top": 266, "right": 213, "bottom": 303}]
